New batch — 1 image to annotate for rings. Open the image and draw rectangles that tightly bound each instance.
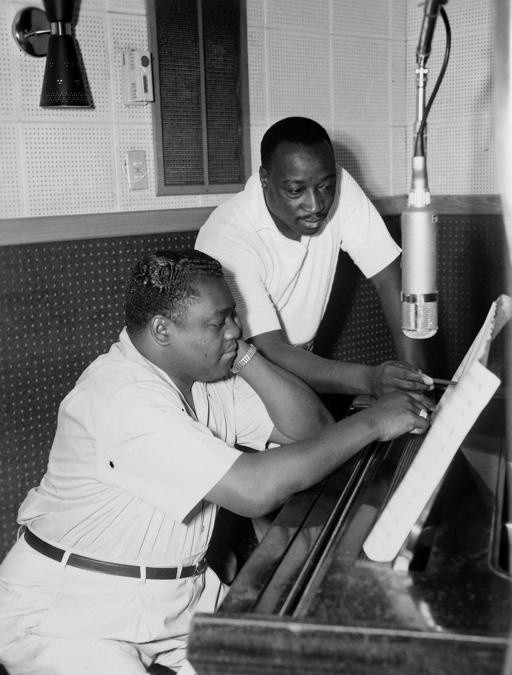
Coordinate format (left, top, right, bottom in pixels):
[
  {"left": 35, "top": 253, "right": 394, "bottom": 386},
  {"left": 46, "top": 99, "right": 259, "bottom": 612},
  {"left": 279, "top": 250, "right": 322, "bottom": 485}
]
[{"left": 418, "top": 409, "right": 428, "bottom": 420}]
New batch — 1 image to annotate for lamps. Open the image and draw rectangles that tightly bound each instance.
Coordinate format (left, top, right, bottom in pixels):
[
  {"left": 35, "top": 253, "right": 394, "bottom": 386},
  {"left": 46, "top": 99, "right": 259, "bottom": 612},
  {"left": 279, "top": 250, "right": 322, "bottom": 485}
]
[{"left": 14, "top": 0, "right": 97, "bottom": 109}]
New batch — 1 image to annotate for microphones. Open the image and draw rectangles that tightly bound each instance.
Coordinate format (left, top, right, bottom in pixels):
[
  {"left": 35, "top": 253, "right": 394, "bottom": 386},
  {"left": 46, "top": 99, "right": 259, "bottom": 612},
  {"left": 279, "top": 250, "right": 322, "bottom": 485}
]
[{"left": 400, "top": 135, "right": 439, "bottom": 340}]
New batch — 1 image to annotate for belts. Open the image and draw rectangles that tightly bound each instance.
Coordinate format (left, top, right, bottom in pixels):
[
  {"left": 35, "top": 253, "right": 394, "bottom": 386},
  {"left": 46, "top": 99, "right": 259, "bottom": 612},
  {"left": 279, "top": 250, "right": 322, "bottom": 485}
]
[{"left": 22, "top": 527, "right": 210, "bottom": 580}]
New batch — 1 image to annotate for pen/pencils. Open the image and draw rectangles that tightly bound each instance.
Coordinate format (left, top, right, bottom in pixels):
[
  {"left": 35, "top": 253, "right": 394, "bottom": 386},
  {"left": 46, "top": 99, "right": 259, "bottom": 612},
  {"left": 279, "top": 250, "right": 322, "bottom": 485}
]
[{"left": 433, "top": 378, "right": 457, "bottom": 385}]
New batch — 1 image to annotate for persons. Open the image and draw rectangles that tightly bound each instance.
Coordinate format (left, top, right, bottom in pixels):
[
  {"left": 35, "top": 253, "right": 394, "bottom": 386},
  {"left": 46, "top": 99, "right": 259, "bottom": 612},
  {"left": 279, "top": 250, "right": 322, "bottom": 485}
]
[
  {"left": 0, "top": 246, "right": 431, "bottom": 675},
  {"left": 194, "top": 116, "right": 435, "bottom": 545}
]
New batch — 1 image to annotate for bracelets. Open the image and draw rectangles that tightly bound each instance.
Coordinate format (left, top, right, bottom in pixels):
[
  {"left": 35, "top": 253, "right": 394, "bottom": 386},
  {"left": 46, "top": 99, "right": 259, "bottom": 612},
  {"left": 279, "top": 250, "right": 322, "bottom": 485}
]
[{"left": 229, "top": 343, "right": 258, "bottom": 374}]
